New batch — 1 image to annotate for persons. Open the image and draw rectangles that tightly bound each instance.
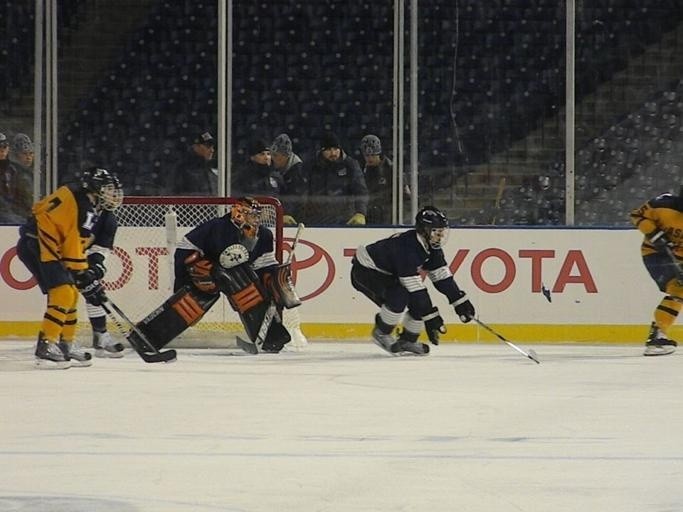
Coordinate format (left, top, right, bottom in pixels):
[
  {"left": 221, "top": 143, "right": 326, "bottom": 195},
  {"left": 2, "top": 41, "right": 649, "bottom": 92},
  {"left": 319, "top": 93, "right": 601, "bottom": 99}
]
[
  {"left": 246, "top": 222, "right": 278, "bottom": 278},
  {"left": 11, "top": 133, "right": 35, "bottom": 170},
  {"left": 16, "top": 166, "right": 124, "bottom": 366},
  {"left": 128, "top": 196, "right": 291, "bottom": 352},
  {"left": 628, "top": 192, "right": 683, "bottom": 356},
  {"left": 166, "top": 127, "right": 411, "bottom": 226},
  {"left": 350, "top": 205, "right": 476, "bottom": 354},
  {"left": 0, "top": 132, "right": 36, "bottom": 225},
  {"left": 82, "top": 209, "right": 126, "bottom": 358}
]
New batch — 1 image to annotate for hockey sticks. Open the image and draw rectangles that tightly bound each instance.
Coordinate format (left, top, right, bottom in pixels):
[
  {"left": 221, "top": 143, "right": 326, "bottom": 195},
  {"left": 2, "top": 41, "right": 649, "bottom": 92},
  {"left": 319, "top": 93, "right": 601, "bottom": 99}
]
[
  {"left": 104, "top": 296, "right": 178, "bottom": 364},
  {"left": 468, "top": 314, "right": 541, "bottom": 364},
  {"left": 98, "top": 304, "right": 176, "bottom": 363},
  {"left": 237, "top": 223, "right": 305, "bottom": 353}
]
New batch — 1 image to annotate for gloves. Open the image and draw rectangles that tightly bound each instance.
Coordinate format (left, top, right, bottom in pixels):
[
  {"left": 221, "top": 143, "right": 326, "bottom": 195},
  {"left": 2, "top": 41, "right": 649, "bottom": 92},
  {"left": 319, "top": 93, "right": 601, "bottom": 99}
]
[
  {"left": 346, "top": 213, "right": 366, "bottom": 225},
  {"left": 645, "top": 227, "right": 677, "bottom": 256},
  {"left": 71, "top": 264, "right": 107, "bottom": 306},
  {"left": 282, "top": 216, "right": 297, "bottom": 225},
  {"left": 449, "top": 294, "right": 475, "bottom": 322},
  {"left": 421, "top": 306, "right": 447, "bottom": 345}
]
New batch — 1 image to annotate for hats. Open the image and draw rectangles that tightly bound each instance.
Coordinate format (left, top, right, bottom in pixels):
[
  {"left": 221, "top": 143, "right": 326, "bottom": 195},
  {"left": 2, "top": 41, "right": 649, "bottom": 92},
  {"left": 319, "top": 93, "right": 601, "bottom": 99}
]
[
  {"left": 244, "top": 134, "right": 270, "bottom": 156},
  {"left": 319, "top": 130, "right": 341, "bottom": 152},
  {"left": 187, "top": 130, "right": 215, "bottom": 146},
  {"left": 360, "top": 135, "right": 382, "bottom": 156},
  {"left": 269, "top": 134, "right": 292, "bottom": 158}
]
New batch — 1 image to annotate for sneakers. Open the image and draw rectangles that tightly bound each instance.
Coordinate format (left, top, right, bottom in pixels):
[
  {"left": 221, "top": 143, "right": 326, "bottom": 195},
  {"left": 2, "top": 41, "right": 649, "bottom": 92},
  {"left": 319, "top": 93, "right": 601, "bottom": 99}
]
[
  {"left": 644, "top": 322, "right": 676, "bottom": 346},
  {"left": 372, "top": 327, "right": 403, "bottom": 353},
  {"left": 396, "top": 327, "right": 429, "bottom": 353},
  {"left": 35, "top": 329, "right": 125, "bottom": 360}
]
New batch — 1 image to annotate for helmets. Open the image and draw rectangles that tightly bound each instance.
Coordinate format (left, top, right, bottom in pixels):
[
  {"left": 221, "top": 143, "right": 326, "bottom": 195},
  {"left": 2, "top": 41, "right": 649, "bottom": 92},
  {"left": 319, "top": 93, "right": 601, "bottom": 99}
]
[
  {"left": 415, "top": 206, "right": 449, "bottom": 250},
  {"left": 110, "top": 174, "right": 124, "bottom": 212},
  {"left": 81, "top": 168, "right": 114, "bottom": 208},
  {"left": 231, "top": 196, "right": 264, "bottom": 231}
]
